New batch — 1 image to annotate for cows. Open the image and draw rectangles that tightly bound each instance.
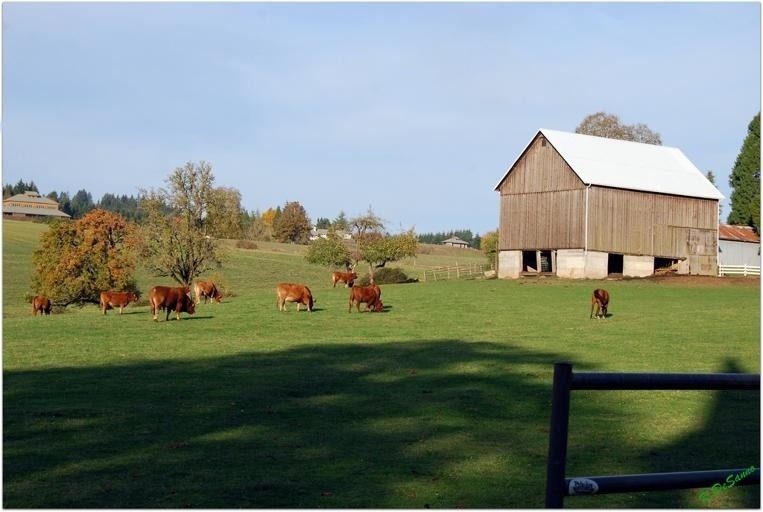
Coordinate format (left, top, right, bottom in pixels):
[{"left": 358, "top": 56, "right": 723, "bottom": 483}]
[
  {"left": 32, "top": 295, "right": 53, "bottom": 317},
  {"left": 347, "top": 284, "right": 384, "bottom": 313},
  {"left": 99, "top": 291, "right": 138, "bottom": 315},
  {"left": 590, "top": 288, "right": 610, "bottom": 321},
  {"left": 149, "top": 286, "right": 196, "bottom": 321},
  {"left": 331, "top": 271, "right": 358, "bottom": 289},
  {"left": 194, "top": 280, "right": 223, "bottom": 304},
  {"left": 276, "top": 283, "right": 317, "bottom": 313}
]
